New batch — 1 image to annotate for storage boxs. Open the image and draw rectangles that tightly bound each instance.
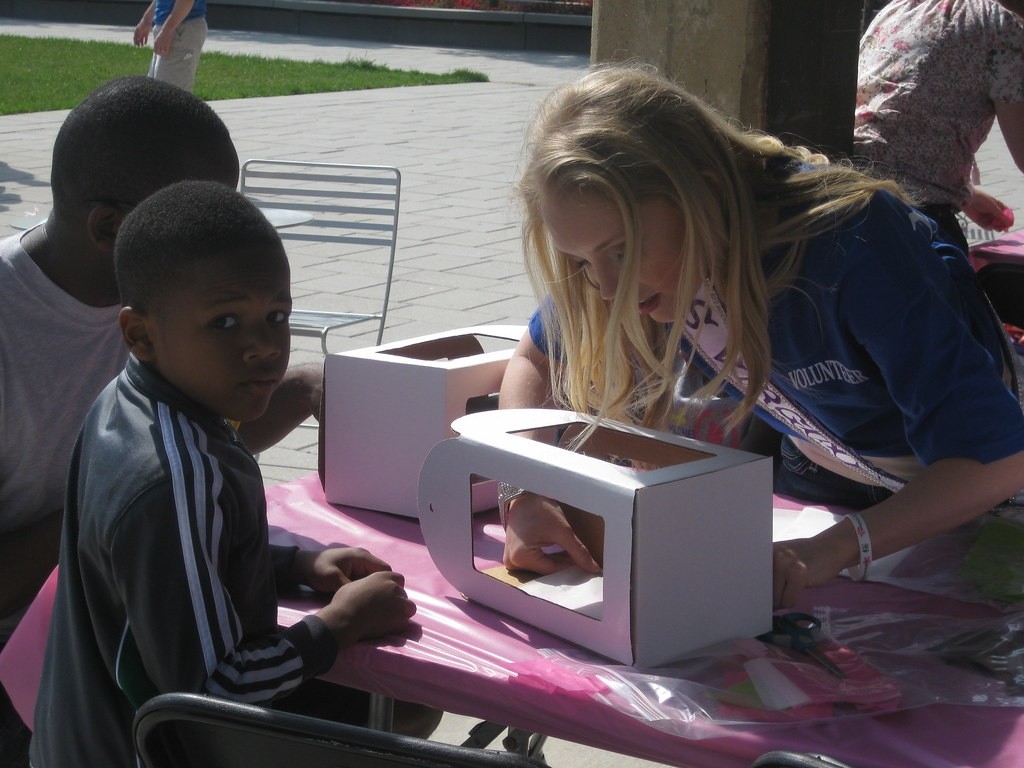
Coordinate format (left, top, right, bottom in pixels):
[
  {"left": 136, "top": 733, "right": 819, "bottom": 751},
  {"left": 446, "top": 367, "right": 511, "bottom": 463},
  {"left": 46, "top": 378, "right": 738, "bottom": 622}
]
[
  {"left": 317, "top": 325, "right": 528, "bottom": 517},
  {"left": 417, "top": 409, "right": 774, "bottom": 668}
]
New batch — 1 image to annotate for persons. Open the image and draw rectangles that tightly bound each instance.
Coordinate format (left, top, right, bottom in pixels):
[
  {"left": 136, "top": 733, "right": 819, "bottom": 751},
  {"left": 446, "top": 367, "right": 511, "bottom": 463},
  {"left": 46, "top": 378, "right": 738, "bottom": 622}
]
[
  {"left": 853, "top": 0, "right": 1024, "bottom": 258},
  {"left": 29, "top": 182, "right": 441, "bottom": 768},
  {"left": 0, "top": 76, "right": 324, "bottom": 642},
  {"left": 494, "top": 63, "right": 1024, "bottom": 612},
  {"left": 133, "top": 0, "right": 209, "bottom": 94}
]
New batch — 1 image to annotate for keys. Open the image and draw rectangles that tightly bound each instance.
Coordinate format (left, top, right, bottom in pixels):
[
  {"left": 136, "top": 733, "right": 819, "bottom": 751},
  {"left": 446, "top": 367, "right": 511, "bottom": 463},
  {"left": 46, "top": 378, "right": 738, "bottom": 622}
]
[{"left": 770, "top": 613, "right": 843, "bottom": 678}]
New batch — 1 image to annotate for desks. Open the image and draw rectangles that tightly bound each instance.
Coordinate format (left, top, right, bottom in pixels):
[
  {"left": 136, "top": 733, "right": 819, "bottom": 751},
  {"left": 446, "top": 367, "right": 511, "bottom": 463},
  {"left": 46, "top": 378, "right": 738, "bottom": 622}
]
[
  {"left": 969, "top": 228, "right": 1024, "bottom": 353},
  {"left": 0, "top": 471, "right": 1024, "bottom": 768}
]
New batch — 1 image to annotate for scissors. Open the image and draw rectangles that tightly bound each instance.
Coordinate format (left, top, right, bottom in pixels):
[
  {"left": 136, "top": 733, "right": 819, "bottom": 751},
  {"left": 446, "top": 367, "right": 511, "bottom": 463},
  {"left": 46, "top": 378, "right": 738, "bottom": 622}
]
[{"left": 755, "top": 612, "right": 843, "bottom": 676}]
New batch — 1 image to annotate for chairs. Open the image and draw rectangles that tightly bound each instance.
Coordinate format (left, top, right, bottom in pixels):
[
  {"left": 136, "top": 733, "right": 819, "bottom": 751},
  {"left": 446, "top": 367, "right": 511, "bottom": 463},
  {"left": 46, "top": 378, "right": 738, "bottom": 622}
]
[
  {"left": 131, "top": 691, "right": 549, "bottom": 768},
  {"left": 239, "top": 159, "right": 402, "bottom": 462},
  {"left": 976, "top": 261, "right": 1024, "bottom": 330}
]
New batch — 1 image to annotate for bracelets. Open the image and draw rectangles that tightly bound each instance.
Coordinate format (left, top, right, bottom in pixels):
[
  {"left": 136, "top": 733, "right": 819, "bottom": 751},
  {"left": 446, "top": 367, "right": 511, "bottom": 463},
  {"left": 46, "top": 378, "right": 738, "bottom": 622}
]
[
  {"left": 498, "top": 481, "right": 525, "bottom": 532},
  {"left": 843, "top": 513, "right": 873, "bottom": 581}
]
